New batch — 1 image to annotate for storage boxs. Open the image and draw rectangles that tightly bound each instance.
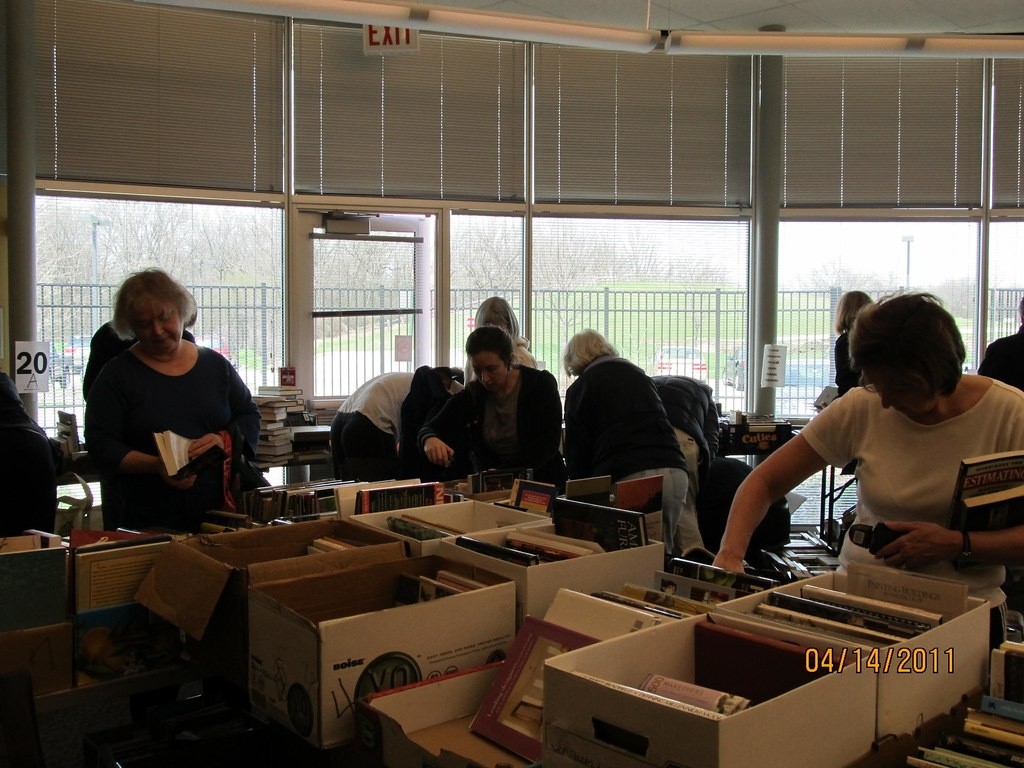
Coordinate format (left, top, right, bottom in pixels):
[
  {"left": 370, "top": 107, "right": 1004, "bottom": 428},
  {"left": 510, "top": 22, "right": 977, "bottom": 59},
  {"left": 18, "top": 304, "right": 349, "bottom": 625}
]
[
  {"left": 729, "top": 417, "right": 793, "bottom": 455},
  {"left": 134, "top": 480, "right": 993, "bottom": 768}
]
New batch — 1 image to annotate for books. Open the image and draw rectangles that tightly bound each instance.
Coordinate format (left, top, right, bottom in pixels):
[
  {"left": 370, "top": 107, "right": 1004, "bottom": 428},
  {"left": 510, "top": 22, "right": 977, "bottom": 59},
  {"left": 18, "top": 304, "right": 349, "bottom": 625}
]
[
  {"left": 50, "top": 411, "right": 83, "bottom": 459},
  {"left": 153, "top": 428, "right": 230, "bottom": 479},
  {"left": 245, "top": 385, "right": 345, "bottom": 467},
  {"left": 0, "top": 452, "right": 1024, "bottom": 767},
  {"left": 814, "top": 386, "right": 841, "bottom": 411}
]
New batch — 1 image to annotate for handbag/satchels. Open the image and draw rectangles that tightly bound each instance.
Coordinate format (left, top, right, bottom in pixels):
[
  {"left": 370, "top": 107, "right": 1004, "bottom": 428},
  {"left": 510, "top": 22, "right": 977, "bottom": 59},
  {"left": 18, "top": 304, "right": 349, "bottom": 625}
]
[{"left": 239, "top": 456, "right": 271, "bottom": 489}]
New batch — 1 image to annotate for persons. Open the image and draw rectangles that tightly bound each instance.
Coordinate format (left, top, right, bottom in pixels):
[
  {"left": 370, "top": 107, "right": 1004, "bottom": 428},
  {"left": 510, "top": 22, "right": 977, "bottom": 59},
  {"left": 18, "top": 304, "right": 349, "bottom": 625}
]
[
  {"left": 0, "top": 371, "right": 55, "bottom": 569},
  {"left": 328, "top": 295, "right": 720, "bottom": 559},
  {"left": 82, "top": 269, "right": 261, "bottom": 538},
  {"left": 817, "top": 291, "right": 1024, "bottom": 474},
  {"left": 712, "top": 293, "right": 1023, "bottom": 648}
]
